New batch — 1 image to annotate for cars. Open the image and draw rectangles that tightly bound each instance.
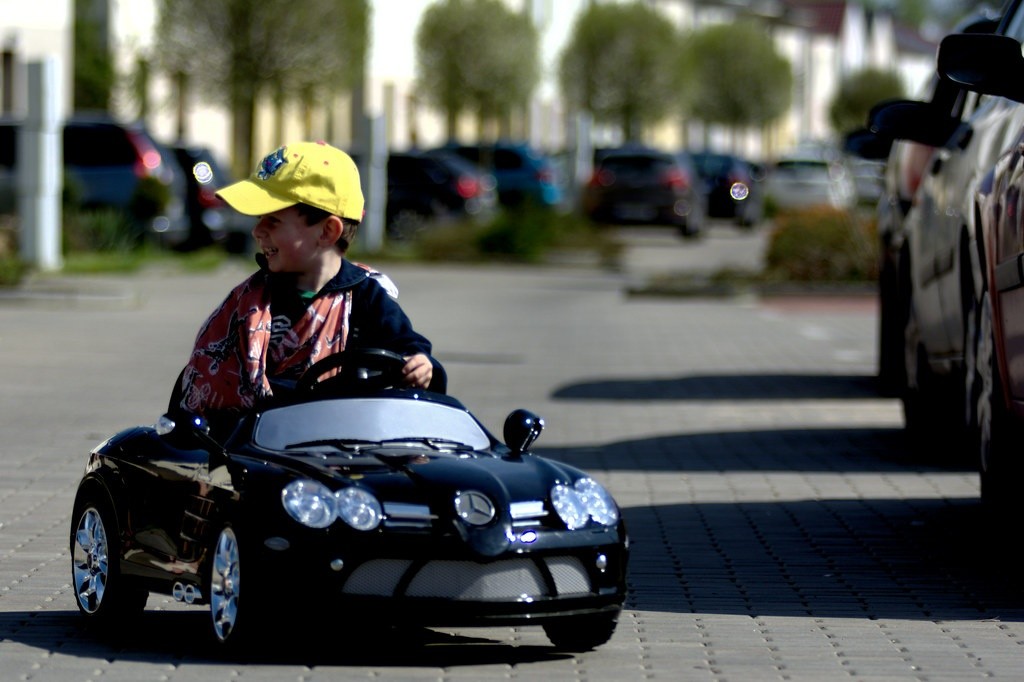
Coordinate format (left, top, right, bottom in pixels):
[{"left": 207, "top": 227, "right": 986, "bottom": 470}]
[
  {"left": 587, "top": 149, "right": 701, "bottom": 238},
  {"left": 69, "top": 349, "right": 632, "bottom": 652},
  {"left": 346, "top": 152, "right": 498, "bottom": 241},
  {"left": 687, "top": 154, "right": 766, "bottom": 228},
  {"left": 166, "top": 147, "right": 229, "bottom": 254},
  {"left": 0, "top": 118, "right": 174, "bottom": 245},
  {"left": 843, "top": 0, "right": 1024, "bottom": 523},
  {"left": 762, "top": 150, "right": 885, "bottom": 218},
  {"left": 424, "top": 144, "right": 582, "bottom": 256}
]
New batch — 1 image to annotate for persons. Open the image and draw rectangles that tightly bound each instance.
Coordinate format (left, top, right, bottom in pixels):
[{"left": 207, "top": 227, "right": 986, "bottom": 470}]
[{"left": 181, "top": 140, "right": 447, "bottom": 443}]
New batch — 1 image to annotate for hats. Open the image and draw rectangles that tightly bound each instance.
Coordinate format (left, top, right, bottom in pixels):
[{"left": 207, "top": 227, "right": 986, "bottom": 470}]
[{"left": 215, "top": 140, "right": 365, "bottom": 223}]
